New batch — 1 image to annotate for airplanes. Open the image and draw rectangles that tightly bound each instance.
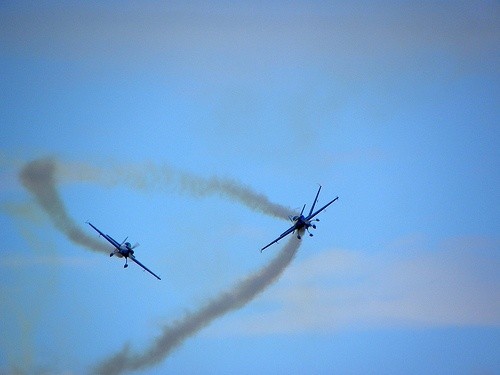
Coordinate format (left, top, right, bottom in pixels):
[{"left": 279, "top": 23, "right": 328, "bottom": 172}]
[
  {"left": 259, "top": 186, "right": 339, "bottom": 249},
  {"left": 85, "top": 221, "right": 163, "bottom": 285}
]
